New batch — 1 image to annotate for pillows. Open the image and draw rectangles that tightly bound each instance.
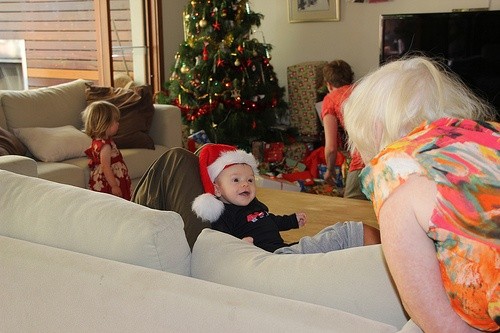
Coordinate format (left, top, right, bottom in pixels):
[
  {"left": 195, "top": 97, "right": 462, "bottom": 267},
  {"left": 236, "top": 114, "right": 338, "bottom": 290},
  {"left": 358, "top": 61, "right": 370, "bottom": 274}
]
[
  {"left": 0, "top": 127, "right": 31, "bottom": 156},
  {"left": 13, "top": 123, "right": 94, "bottom": 162},
  {"left": 83, "top": 81, "right": 156, "bottom": 150}
]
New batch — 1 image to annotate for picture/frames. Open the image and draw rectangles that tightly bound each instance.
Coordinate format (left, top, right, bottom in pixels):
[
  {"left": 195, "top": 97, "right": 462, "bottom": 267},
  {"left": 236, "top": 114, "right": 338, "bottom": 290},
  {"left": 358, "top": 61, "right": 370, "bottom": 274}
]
[{"left": 286, "top": 0, "right": 341, "bottom": 23}]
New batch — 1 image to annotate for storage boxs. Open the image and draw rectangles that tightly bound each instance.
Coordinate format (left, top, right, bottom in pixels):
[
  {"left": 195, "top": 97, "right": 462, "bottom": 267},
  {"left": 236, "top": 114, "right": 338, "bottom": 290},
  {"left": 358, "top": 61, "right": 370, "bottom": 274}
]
[{"left": 253, "top": 60, "right": 350, "bottom": 188}]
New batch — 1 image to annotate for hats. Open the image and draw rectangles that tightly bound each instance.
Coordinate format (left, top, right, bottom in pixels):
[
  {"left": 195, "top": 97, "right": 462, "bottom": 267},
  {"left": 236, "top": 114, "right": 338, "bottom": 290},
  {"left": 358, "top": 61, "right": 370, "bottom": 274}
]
[{"left": 191, "top": 143, "right": 257, "bottom": 221}]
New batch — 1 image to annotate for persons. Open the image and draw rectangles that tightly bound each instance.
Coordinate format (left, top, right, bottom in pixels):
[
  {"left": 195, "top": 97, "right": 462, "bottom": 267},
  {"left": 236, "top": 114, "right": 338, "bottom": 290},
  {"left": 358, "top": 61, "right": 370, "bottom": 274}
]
[
  {"left": 79, "top": 102, "right": 132, "bottom": 202},
  {"left": 129, "top": 143, "right": 381, "bottom": 256},
  {"left": 188, "top": 144, "right": 307, "bottom": 253},
  {"left": 321, "top": 60, "right": 368, "bottom": 201},
  {"left": 336, "top": 51, "right": 500, "bottom": 333}
]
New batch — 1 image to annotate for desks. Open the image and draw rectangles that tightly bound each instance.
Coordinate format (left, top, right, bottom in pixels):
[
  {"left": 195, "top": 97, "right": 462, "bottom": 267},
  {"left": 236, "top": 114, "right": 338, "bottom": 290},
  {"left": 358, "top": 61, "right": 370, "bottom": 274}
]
[{"left": 256, "top": 186, "right": 379, "bottom": 244}]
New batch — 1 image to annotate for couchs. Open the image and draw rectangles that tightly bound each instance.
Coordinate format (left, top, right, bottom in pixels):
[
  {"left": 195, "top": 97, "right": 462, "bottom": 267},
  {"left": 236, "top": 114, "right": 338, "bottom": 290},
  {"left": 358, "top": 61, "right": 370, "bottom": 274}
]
[
  {"left": 0, "top": 168, "right": 423, "bottom": 333},
  {"left": 0, "top": 78, "right": 184, "bottom": 188}
]
